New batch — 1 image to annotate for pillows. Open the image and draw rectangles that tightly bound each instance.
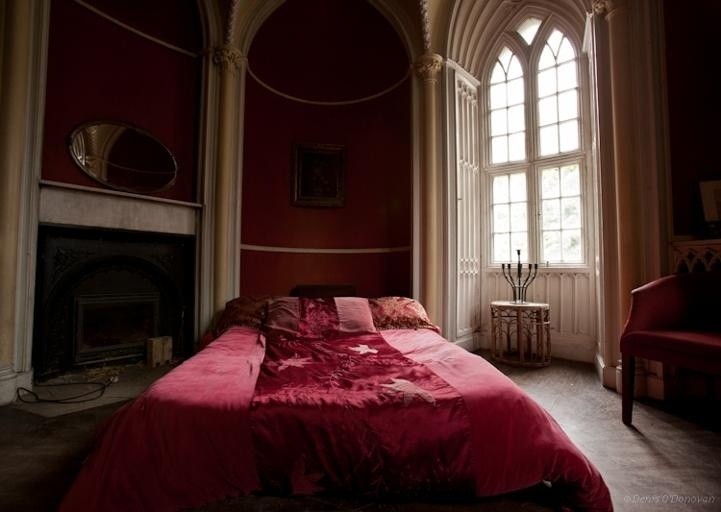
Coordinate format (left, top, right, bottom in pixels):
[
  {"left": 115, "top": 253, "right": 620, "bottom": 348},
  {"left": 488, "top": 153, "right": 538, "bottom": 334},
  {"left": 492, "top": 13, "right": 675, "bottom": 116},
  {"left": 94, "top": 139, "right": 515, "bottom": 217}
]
[
  {"left": 213, "top": 292, "right": 273, "bottom": 335},
  {"left": 370, "top": 295, "right": 442, "bottom": 335},
  {"left": 262, "top": 295, "right": 379, "bottom": 337}
]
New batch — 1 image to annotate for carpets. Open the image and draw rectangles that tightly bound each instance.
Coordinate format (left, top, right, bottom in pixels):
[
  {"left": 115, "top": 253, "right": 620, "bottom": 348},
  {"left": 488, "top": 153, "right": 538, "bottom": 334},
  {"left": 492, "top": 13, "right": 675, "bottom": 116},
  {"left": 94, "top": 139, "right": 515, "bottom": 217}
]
[{"left": 14, "top": 356, "right": 186, "bottom": 421}]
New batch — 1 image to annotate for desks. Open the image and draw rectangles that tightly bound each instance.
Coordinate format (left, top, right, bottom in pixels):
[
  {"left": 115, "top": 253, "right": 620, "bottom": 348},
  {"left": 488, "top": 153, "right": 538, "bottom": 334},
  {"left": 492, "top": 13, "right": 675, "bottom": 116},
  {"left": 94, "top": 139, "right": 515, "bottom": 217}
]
[{"left": 489, "top": 299, "right": 553, "bottom": 369}]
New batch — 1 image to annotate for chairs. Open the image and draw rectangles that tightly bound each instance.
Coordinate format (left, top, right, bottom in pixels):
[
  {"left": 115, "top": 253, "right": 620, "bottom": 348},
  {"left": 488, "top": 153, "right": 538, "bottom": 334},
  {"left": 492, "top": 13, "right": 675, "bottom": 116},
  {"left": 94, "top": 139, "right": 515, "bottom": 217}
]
[{"left": 619, "top": 272, "right": 721, "bottom": 426}]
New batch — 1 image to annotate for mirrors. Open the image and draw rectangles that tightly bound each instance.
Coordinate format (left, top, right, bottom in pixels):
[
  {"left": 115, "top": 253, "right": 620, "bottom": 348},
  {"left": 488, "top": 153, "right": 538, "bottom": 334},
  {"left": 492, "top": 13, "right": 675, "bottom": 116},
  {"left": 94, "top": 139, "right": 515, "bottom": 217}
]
[{"left": 68, "top": 120, "right": 180, "bottom": 195}]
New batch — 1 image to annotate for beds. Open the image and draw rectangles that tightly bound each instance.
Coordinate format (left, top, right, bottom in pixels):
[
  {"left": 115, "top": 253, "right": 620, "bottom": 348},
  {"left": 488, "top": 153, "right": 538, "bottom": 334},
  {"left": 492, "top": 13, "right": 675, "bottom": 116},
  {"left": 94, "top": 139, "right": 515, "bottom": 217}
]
[{"left": 59, "top": 283, "right": 617, "bottom": 509}]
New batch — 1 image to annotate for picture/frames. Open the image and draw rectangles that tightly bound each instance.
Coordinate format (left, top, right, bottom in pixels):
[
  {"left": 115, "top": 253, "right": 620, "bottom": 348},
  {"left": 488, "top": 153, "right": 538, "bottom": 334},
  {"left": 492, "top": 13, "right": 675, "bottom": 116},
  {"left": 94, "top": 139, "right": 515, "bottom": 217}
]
[{"left": 290, "top": 139, "right": 349, "bottom": 209}]
